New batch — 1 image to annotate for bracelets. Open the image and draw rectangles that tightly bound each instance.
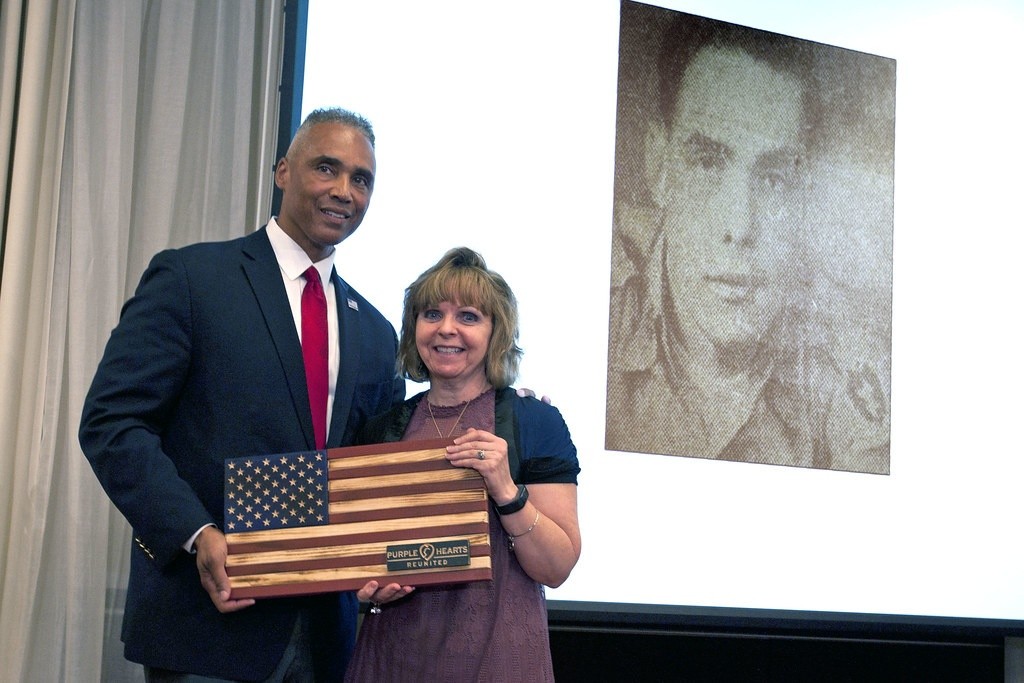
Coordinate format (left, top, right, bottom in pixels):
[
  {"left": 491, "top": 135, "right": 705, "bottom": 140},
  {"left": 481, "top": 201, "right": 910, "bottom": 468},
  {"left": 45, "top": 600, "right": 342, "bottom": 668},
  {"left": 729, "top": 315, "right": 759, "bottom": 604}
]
[{"left": 504, "top": 511, "right": 539, "bottom": 550}]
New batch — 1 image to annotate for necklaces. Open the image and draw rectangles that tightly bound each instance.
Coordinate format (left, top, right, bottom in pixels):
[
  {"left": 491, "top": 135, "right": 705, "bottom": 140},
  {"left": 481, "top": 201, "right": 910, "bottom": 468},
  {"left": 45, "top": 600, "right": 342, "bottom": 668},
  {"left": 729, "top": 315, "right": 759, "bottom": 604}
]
[{"left": 428, "top": 400, "right": 471, "bottom": 438}]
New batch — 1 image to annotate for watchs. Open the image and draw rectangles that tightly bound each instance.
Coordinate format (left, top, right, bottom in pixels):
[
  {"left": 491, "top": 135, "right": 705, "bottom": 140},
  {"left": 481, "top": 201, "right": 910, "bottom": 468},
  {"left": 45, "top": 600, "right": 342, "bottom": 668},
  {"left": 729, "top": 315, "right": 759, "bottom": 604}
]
[{"left": 495, "top": 485, "right": 530, "bottom": 515}]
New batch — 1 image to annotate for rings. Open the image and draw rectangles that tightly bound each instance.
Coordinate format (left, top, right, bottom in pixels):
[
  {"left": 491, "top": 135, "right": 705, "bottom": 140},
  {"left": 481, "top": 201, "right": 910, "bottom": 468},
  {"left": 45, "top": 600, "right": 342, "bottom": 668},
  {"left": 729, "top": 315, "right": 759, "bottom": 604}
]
[
  {"left": 478, "top": 450, "right": 485, "bottom": 459},
  {"left": 370, "top": 598, "right": 383, "bottom": 614}
]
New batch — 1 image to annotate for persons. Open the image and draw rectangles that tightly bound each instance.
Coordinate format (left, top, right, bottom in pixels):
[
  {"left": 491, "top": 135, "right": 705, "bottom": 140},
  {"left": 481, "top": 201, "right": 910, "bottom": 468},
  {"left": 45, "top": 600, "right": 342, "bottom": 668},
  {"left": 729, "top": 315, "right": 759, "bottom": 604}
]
[
  {"left": 603, "top": 15, "right": 892, "bottom": 476},
  {"left": 78, "top": 108, "right": 407, "bottom": 683},
  {"left": 345, "top": 246, "right": 582, "bottom": 683}
]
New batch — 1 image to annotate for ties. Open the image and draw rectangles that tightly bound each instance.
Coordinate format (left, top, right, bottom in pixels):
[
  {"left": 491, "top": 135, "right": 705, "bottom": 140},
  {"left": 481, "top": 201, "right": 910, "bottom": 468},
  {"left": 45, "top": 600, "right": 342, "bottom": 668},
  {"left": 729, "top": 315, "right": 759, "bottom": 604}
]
[{"left": 297, "top": 265, "right": 329, "bottom": 449}]
